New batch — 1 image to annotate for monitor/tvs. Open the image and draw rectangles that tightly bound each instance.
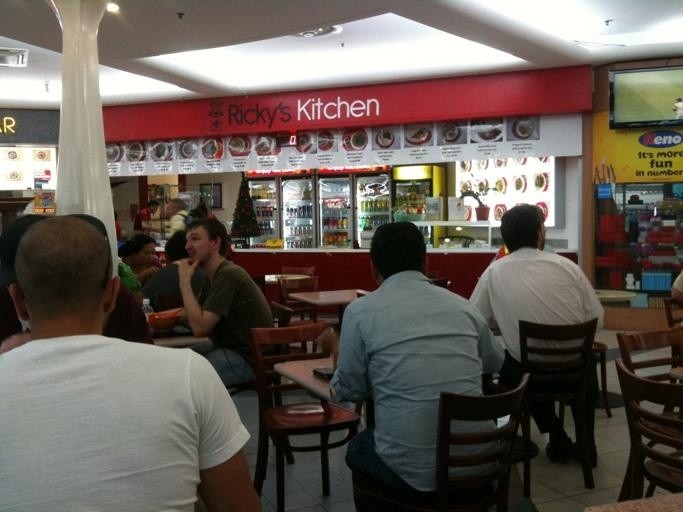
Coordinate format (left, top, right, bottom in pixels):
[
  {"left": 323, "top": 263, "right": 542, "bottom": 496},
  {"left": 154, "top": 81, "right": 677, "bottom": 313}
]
[{"left": 607, "top": 65, "right": 683, "bottom": 129}]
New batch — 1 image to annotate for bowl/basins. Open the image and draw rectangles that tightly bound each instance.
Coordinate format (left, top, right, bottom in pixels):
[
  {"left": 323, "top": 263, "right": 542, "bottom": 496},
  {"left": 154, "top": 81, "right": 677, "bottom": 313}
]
[{"left": 148, "top": 313, "right": 179, "bottom": 333}]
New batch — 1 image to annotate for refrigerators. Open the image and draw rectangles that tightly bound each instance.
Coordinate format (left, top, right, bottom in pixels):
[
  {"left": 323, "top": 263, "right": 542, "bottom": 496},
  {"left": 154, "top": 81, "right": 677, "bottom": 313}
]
[
  {"left": 243, "top": 168, "right": 316, "bottom": 248},
  {"left": 391, "top": 165, "right": 446, "bottom": 247},
  {"left": 316, "top": 165, "right": 390, "bottom": 248}
]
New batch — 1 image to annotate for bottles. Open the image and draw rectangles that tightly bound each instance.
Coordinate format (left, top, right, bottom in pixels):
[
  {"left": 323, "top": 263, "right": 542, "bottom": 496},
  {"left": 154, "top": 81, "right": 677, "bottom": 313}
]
[
  {"left": 285, "top": 237, "right": 311, "bottom": 248},
  {"left": 249, "top": 239, "right": 265, "bottom": 247},
  {"left": 289, "top": 225, "right": 311, "bottom": 235},
  {"left": 360, "top": 199, "right": 388, "bottom": 213},
  {"left": 251, "top": 184, "right": 276, "bottom": 199},
  {"left": 398, "top": 188, "right": 424, "bottom": 200},
  {"left": 322, "top": 233, "right": 347, "bottom": 247},
  {"left": 321, "top": 199, "right": 347, "bottom": 214},
  {"left": 418, "top": 226, "right": 430, "bottom": 244},
  {"left": 357, "top": 183, "right": 388, "bottom": 197},
  {"left": 257, "top": 220, "right": 273, "bottom": 234},
  {"left": 358, "top": 215, "right": 384, "bottom": 231},
  {"left": 285, "top": 203, "right": 311, "bottom": 218},
  {"left": 140, "top": 298, "right": 153, "bottom": 341},
  {"left": 322, "top": 217, "right": 346, "bottom": 229},
  {"left": 401, "top": 203, "right": 425, "bottom": 214},
  {"left": 256, "top": 203, "right": 275, "bottom": 216}
]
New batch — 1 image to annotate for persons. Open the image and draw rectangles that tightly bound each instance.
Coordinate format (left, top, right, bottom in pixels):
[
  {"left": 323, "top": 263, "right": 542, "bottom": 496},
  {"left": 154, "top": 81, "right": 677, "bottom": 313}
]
[
  {"left": 671, "top": 269, "right": 682, "bottom": 302},
  {"left": 671, "top": 95, "right": 683, "bottom": 119},
  {"left": 328, "top": 222, "right": 506, "bottom": 510},
  {"left": 1, "top": 197, "right": 275, "bottom": 512},
  {"left": 467, "top": 204, "right": 604, "bottom": 465}
]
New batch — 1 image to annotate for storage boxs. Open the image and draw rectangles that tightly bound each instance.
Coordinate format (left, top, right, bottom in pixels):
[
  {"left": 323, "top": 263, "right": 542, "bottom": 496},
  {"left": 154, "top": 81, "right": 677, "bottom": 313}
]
[{"left": 590, "top": 181, "right": 682, "bottom": 295}]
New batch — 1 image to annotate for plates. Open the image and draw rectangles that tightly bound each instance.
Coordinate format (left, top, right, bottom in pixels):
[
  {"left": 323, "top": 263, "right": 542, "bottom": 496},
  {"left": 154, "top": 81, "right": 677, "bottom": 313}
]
[
  {"left": 181, "top": 141, "right": 196, "bottom": 158},
  {"left": 512, "top": 159, "right": 526, "bottom": 166},
  {"left": 495, "top": 177, "right": 504, "bottom": 194},
  {"left": 378, "top": 130, "right": 393, "bottom": 147},
  {"left": 476, "top": 179, "right": 486, "bottom": 194},
  {"left": 126, "top": 142, "right": 142, "bottom": 161},
  {"left": 5, "top": 150, "right": 19, "bottom": 161},
  {"left": 34, "top": 151, "right": 47, "bottom": 161},
  {"left": 227, "top": 137, "right": 245, "bottom": 154},
  {"left": 105, "top": 144, "right": 119, "bottom": 163},
  {"left": 494, "top": 207, "right": 502, "bottom": 221},
  {"left": 405, "top": 129, "right": 428, "bottom": 143},
  {"left": 469, "top": 125, "right": 502, "bottom": 143},
  {"left": 493, "top": 158, "right": 507, "bottom": 168},
  {"left": 7, "top": 170, "right": 20, "bottom": 181},
  {"left": 460, "top": 160, "right": 471, "bottom": 172},
  {"left": 478, "top": 160, "right": 488, "bottom": 170},
  {"left": 349, "top": 131, "right": 367, "bottom": 150},
  {"left": 150, "top": 142, "right": 169, "bottom": 161},
  {"left": 441, "top": 123, "right": 459, "bottom": 142},
  {"left": 255, "top": 136, "right": 274, "bottom": 155},
  {"left": 202, "top": 138, "right": 218, "bottom": 158},
  {"left": 512, "top": 177, "right": 526, "bottom": 193},
  {"left": 533, "top": 173, "right": 546, "bottom": 192},
  {"left": 459, "top": 181, "right": 470, "bottom": 192},
  {"left": 516, "top": 119, "right": 533, "bottom": 138}
]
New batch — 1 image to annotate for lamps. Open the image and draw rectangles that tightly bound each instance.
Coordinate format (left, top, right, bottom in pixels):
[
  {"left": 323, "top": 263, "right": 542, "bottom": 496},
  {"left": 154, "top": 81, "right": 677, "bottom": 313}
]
[{"left": 292, "top": 25, "right": 340, "bottom": 40}]
